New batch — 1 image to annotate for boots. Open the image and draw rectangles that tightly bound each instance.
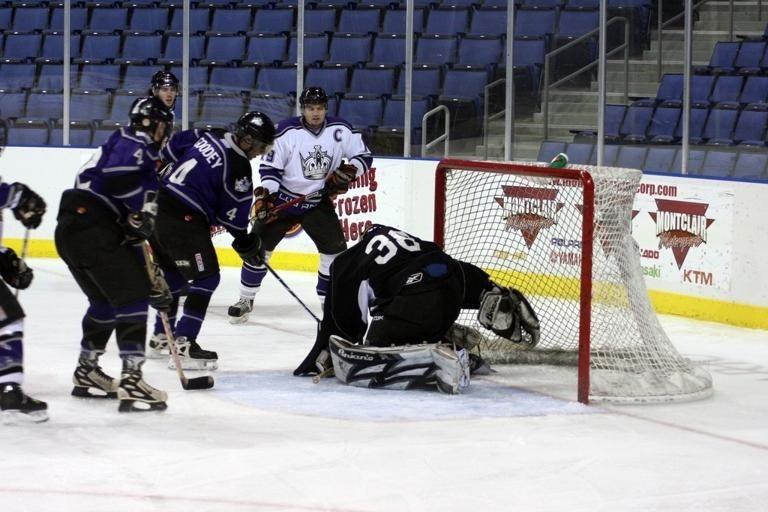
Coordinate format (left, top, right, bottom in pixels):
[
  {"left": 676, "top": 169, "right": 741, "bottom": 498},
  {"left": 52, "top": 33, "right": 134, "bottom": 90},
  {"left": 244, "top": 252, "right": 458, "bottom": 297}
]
[
  {"left": 228, "top": 282, "right": 258, "bottom": 317},
  {"left": 72, "top": 348, "right": 117, "bottom": 392},
  {"left": 117, "top": 354, "right": 168, "bottom": 403}
]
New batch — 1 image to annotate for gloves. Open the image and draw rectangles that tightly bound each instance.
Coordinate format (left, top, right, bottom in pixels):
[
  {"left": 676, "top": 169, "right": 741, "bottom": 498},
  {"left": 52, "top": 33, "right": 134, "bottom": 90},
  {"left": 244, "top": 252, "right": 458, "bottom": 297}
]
[
  {"left": 147, "top": 268, "right": 174, "bottom": 313},
  {"left": 0, "top": 247, "right": 33, "bottom": 289},
  {"left": 232, "top": 233, "right": 266, "bottom": 266},
  {"left": 254, "top": 187, "right": 280, "bottom": 224},
  {"left": 119, "top": 202, "right": 158, "bottom": 245},
  {"left": 326, "top": 160, "right": 358, "bottom": 190},
  {"left": 9, "top": 181, "right": 46, "bottom": 229}
]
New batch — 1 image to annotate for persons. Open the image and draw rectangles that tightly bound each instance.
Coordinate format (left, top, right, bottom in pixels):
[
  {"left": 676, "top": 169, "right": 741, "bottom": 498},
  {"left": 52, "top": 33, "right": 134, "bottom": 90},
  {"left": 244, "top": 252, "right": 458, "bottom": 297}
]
[
  {"left": 0, "top": 119, "right": 49, "bottom": 412},
  {"left": 146, "top": 69, "right": 181, "bottom": 143},
  {"left": 55, "top": 94, "right": 175, "bottom": 404},
  {"left": 228, "top": 87, "right": 373, "bottom": 317},
  {"left": 153, "top": 110, "right": 277, "bottom": 360},
  {"left": 293, "top": 223, "right": 540, "bottom": 395}
]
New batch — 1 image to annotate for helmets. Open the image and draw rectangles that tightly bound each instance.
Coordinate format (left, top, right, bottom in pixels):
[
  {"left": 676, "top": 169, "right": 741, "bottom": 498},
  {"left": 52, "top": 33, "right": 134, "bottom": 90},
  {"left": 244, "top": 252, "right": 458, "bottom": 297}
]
[
  {"left": 149, "top": 71, "right": 179, "bottom": 111},
  {"left": 298, "top": 87, "right": 329, "bottom": 127},
  {"left": 237, "top": 110, "right": 276, "bottom": 160},
  {"left": 130, "top": 96, "right": 175, "bottom": 150}
]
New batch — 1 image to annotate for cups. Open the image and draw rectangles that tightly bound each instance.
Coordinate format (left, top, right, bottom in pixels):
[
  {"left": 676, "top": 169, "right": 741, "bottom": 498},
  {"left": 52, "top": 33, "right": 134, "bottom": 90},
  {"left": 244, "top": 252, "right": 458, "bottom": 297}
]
[{"left": 549, "top": 153, "right": 569, "bottom": 169}]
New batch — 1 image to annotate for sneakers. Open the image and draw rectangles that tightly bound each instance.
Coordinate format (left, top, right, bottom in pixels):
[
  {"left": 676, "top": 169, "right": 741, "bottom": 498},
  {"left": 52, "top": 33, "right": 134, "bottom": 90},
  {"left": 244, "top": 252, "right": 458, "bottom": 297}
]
[
  {"left": 0, "top": 381, "right": 47, "bottom": 412},
  {"left": 149, "top": 333, "right": 171, "bottom": 349},
  {"left": 169, "top": 336, "right": 217, "bottom": 359}
]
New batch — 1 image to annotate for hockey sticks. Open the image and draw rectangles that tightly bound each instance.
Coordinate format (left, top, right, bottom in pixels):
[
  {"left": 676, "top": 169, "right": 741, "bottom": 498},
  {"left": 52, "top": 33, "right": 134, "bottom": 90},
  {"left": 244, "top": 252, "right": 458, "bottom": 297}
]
[{"left": 142, "top": 240, "right": 214, "bottom": 390}]
